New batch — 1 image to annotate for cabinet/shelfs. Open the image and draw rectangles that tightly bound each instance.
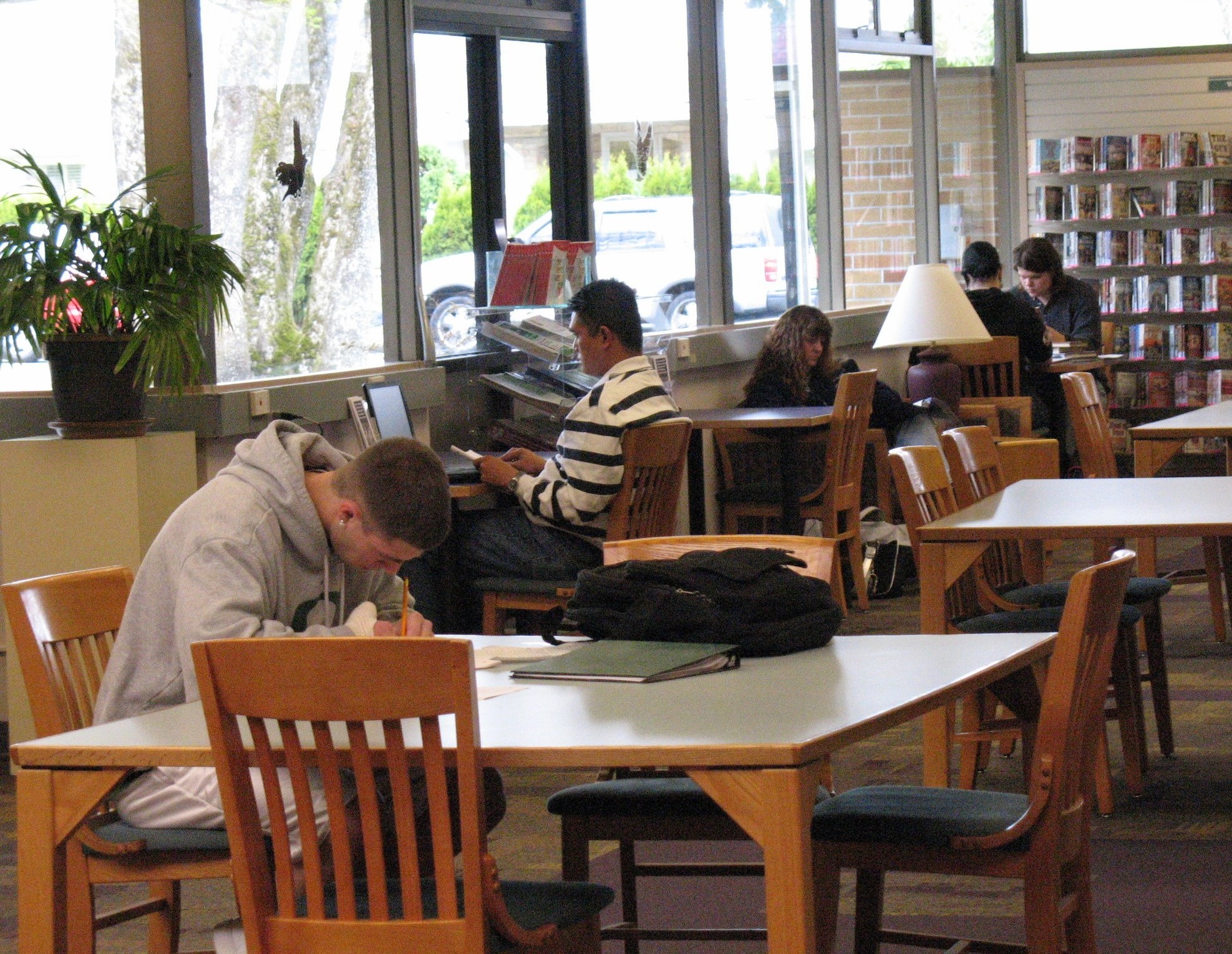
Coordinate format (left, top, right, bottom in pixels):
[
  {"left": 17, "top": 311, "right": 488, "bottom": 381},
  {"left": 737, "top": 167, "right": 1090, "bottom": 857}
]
[{"left": 1030, "top": 168, "right": 1232, "bottom": 473}]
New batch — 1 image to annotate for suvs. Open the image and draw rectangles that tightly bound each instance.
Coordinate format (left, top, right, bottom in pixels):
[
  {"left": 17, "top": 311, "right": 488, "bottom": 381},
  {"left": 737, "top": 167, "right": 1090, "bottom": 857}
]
[{"left": 420, "top": 194, "right": 820, "bottom": 358}]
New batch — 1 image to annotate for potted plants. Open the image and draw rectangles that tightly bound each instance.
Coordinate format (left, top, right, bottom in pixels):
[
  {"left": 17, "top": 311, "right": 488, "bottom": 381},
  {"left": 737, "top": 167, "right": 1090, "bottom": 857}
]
[{"left": 0, "top": 147, "right": 249, "bottom": 438}]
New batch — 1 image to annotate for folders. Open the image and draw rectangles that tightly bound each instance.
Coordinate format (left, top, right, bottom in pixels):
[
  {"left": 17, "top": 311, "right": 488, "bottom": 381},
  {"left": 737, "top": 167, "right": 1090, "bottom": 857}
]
[{"left": 508, "top": 640, "right": 741, "bottom": 684}]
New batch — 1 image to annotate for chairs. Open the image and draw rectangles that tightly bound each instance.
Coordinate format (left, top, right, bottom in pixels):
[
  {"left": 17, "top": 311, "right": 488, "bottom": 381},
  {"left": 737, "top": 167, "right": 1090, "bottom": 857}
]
[{"left": 0, "top": 321, "right": 1232, "bottom": 954}]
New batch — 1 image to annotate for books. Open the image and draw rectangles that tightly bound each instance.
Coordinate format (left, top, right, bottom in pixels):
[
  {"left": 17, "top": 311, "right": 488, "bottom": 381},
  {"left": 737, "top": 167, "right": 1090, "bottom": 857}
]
[
  {"left": 1107, "top": 418, "right": 1223, "bottom": 455},
  {"left": 490, "top": 241, "right": 595, "bottom": 305},
  {"left": 479, "top": 315, "right": 603, "bottom": 452},
  {"left": 508, "top": 640, "right": 742, "bottom": 684},
  {"left": 1027, "top": 131, "right": 1232, "bottom": 174},
  {"left": 1031, "top": 227, "right": 1232, "bottom": 269},
  {"left": 1114, "top": 369, "right": 1232, "bottom": 409},
  {"left": 450, "top": 445, "right": 483, "bottom": 460},
  {"left": 1112, "top": 322, "right": 1232, "bottom": 361},
  {"left": 1036, "top": 178, "right": 1232, "bottom": 221},
  {"left": 1079, "top": 274, "right": 1232, "bottom": 314}
]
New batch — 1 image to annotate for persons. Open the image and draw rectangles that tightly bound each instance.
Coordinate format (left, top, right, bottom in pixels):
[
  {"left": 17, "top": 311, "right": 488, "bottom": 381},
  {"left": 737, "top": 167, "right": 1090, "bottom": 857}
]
[
  {"left": 736, "top": 305, "right": 903, "bottom": 536},
  {"left": 905, "top": 241, "right": 1067, "bottom": 464},
  {"left": 92, "top": 419, "right": 504, "bottom": 954},
  {"left": 1006, "top": 238, "right": 1102, "bottom": 355},
  {"left": 456, "top": 280, "right": 680, "bottom": 634}
]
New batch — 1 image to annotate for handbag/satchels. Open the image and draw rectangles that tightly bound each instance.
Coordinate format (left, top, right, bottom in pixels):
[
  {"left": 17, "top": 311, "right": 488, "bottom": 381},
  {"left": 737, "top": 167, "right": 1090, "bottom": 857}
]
[
  {"left": 540, "top": 546, "right": 842, "bottom": 657},
  {"left": 804, "top": 506, "right": 907, "bottom": 595}
]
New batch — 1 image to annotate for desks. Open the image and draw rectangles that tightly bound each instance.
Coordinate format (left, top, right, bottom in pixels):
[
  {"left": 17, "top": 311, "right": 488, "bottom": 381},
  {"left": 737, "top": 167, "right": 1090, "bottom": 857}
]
[
  {"left": 1043, "top": 359, "right": 1102, "bottom": 372},
  {"left": 1128, "top": 397, "right": 1232, "bottom": 654},
  {"left": 442, "top": 482, "right": 494, "bottom": 498},
  {"left": 677, "top": 408, "right": 835, "bottom": 536},
  {"left": 915, "top": 476, "right": 1232, "bottom": 789},
  {"left": 9, "top": 631, "right": 1058, "bottom": 954}
]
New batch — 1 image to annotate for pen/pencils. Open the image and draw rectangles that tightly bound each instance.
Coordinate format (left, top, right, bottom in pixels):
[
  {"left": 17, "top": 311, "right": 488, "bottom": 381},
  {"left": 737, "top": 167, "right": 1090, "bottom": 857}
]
[{"left": 401, "top": 576, "right": 408, "bottom": 637}]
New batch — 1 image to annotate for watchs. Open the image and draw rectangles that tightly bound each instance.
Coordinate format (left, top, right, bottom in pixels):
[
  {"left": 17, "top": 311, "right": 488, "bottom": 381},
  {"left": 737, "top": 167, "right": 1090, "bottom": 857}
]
[{"left": 506, "top": 471, "right": 526, "bottom": 494}]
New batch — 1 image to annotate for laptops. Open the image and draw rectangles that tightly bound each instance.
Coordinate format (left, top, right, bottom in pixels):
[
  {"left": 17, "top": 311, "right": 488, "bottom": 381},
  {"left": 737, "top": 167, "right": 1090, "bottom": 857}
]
[{"left": 362, "top": 379, "right": 482, "bottom": 482}]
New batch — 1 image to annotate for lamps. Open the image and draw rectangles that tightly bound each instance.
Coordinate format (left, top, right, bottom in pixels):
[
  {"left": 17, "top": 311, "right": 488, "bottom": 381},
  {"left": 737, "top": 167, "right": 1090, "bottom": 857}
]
[{"left": 871, "top": 261, "right": 992, "bottom": 416}]
